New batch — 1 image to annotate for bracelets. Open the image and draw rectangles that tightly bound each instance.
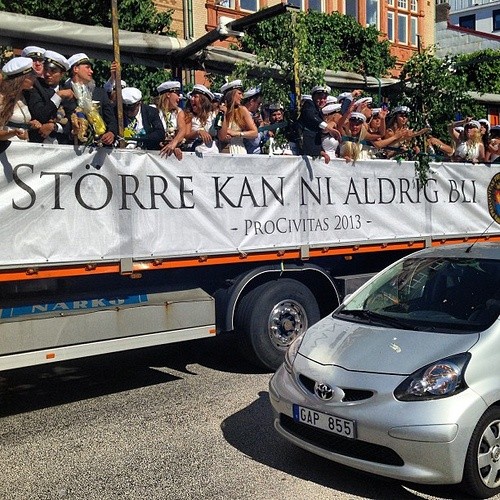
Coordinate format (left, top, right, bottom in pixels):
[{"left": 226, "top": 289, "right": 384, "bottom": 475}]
[{"left": 240, "top": 131, "right": 244, "bottom": 138}]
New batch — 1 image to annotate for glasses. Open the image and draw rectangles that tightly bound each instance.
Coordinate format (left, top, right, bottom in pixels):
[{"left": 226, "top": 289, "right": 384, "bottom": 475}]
[{"left": 396, "top": 113, "right": 409, "bottom": 117}]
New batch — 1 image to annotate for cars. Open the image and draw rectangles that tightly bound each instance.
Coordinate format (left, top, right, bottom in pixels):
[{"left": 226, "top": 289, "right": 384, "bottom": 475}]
[{"left": 267, "top": 242, "right": 500, "bottom": 499}]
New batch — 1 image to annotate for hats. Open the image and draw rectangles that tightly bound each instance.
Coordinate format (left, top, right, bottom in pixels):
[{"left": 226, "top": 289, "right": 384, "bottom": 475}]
[
  {"left": 338, "top": 92, "right": 352, "bottom": 103},
  {"left": 45, "top": 50, "right": 70, "bottom": 73},
  {"left": 371, "top": 108, "right": 381, "bottom": 118},
  {"left": 311, "top": 85, "right": 332, "bottom": 95},
  {"left": 269, "top": 103, "right": 284, "bottom": 114},
  {"left": 353, "top": 97, "right": 373, "bottom": 109},
  {"left": 188, "top": 84, "right": 215, "bottom": 101},
  {"left": 322, "top": 104, "right": 342, "bottom": 115},
  {"left": 213, "top": 93, "right": 224, "bottom": 103},
  {"left": 66, "top": 53, "right": 94, "bottom": 73},
  {"left": 326, "top": 95, "right": 337, "bottom": 104},
  {"left": 301, "top": 95, "right": 314, "bottom": 101},
  {"left": 111, "top": 80, "right": 126, "bottom": 91},
  {"left": 20, "top": 45, "right": 46, "bottom": 61},
  {"left": 186, "top": 91, "right": 194, "bottom": 100},
  {"left": 348, "top": 112, "right": 367, "bottom": 123},
  {"left": 2, "top": 56, "right": 34, "bottom": 78},
  {"left": 157, "top": 81, "right": 181, "bottom": 95},
  {"left": 220, "top": 80, "right": 245, "bottom": 95},
  {"left": 243, "top": 83, "right": 261, "bottom": 99},
  {"left": 121, "top": 87, "right": 142, "bottom": 107},
  {"left": 454, "top": 118, "right": 500, "bottom": 138},
  {"left": 392, "top": 105, "right": 411, "bottom": 115}
]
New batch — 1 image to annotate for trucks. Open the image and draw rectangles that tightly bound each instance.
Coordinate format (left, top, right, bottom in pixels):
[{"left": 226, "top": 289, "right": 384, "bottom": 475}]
[{"left": 1, "top": 10, "right": 500, "bottom": 372}]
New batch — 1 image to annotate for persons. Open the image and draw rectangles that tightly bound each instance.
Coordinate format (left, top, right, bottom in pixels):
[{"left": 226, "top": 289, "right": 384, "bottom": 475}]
[{"left": 0, "top": 47, "right": 500, "bottom": 165}]
